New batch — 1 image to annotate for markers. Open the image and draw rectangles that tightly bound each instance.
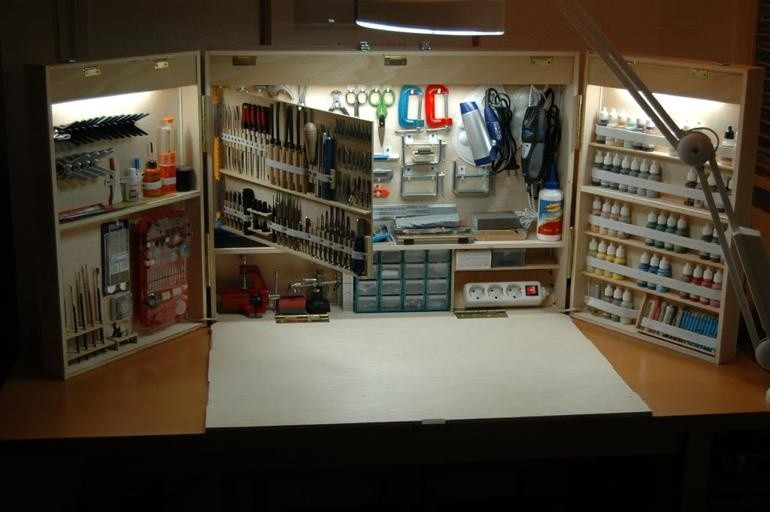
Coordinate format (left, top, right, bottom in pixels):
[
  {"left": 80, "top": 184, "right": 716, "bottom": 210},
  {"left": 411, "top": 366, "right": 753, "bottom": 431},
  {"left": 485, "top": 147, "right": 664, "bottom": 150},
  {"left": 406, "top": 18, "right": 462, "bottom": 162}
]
[
  {"left": 320, "top": 134, "right": 328, "bottom": 199},
  {"left": 224, "top": 187, "right": 271, "bottom": 241},
  {"left": 324, "top": 137, "right": 335, "bottom": 201}
]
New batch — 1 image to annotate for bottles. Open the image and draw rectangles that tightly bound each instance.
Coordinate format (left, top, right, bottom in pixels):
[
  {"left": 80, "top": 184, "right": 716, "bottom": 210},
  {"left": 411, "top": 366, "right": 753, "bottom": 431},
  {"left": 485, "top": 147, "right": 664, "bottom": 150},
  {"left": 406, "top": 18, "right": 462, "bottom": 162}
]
[
  {"left": 459, "top": 101, "right": 496, "bottom": 167},
  {"left": 682, "top": 167, "right": 736, "bottom": 216},
  {"left": 699, "top": 224, "right": 724, "bottom": 263},
  {"left": 644, "top": 209, "right": 690, "bottom": 255},
  {"left": 603, "top": 284, "right": 632, "bottom": 325},
  {"left": 586, "top": 238, "right": 627, "bottom": 281},
  {"left": 719, "top": 126, "right": 736, "bottom": 168},
  {"left": 142, "top": 160, "right": 164, "bottom": 198},
  {"left": 125, "top": 168, "right": 142, "bottom": 203},
  {"left": 590, "top": 150, "right": 662, "bottom": 198},
  {"left": 536, "top": 163, "right": 563, "bottom": 242},
  {"left": 667, "top": 116, "right": 681, "bottom": 157},
  {"left": 589, "top": 198, "right": 631, "bottom": 240},
  {"left": 636, "top": 251, "right": 672, "bottom": 295},
  {"left": 596, "top": 107, "right": 658, "bottom": 152},
  {"left": 679, "top": 262, "right": 723, "bottom": 309}
]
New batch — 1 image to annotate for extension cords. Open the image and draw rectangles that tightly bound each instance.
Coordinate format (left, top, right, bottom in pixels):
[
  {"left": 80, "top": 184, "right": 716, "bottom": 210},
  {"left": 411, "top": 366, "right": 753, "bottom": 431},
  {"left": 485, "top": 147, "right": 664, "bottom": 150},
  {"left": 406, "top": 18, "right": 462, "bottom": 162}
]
[{"left": 462, "top": 280, "right": 550, "bottom": 308}]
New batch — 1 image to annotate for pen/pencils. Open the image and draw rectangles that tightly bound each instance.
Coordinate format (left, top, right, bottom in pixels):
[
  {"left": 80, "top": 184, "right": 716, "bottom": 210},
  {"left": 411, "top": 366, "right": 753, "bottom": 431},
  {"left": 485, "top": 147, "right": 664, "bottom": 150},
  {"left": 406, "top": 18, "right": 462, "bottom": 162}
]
[{"left": 305, "top": 217, "right": 312, "bottom": 254}]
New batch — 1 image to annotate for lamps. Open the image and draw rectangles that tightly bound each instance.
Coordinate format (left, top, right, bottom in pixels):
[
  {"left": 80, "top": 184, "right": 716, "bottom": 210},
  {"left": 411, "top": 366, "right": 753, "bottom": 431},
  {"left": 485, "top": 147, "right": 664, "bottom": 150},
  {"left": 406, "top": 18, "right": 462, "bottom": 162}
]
[{"left": 356, "top": 1, "right": 760, "bottom": 365}]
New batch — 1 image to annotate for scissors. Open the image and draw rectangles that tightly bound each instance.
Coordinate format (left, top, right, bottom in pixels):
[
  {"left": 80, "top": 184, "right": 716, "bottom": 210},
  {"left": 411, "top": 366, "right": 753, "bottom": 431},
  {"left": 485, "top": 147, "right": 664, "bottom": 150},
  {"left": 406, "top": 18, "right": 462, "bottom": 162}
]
[
  {"left": 369, "top": 89, "right": 396, "bottom": 147},
  {"left": 346, "top": 89, "right": 367, "bottom": 116}
]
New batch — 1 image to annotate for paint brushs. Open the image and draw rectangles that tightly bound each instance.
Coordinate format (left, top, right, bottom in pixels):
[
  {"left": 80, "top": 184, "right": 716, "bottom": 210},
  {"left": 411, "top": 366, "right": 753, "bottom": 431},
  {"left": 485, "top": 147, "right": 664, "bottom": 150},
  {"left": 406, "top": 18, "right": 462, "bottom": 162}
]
[
  {"left": 311, "top": 207, "right": 356, "bottom": 270},
  {"left": 69, "top": 265, "right": 108, "bottom": 364},
  {"left": 271, "top": 190, "right": 304, "bottom": 252}
]
